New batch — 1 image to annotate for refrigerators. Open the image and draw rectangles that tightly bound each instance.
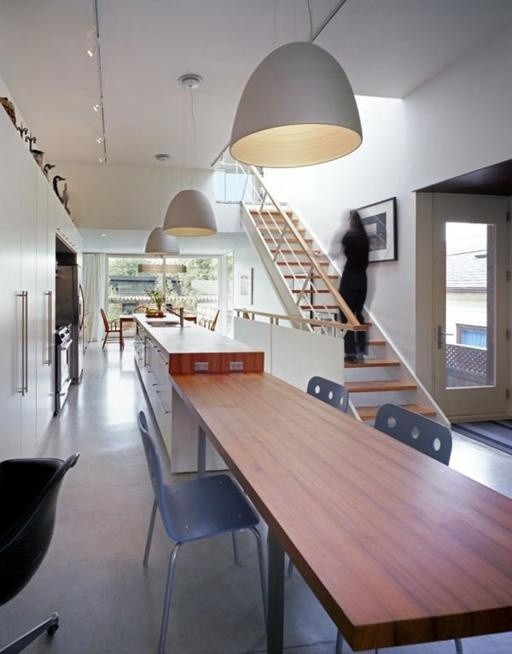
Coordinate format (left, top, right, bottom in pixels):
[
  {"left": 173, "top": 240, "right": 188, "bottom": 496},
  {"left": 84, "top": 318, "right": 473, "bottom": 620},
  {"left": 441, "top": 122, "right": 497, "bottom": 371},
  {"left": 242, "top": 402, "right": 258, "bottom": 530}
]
[{"left": 56, "top": 264, "right": 84, "bottom": 385}]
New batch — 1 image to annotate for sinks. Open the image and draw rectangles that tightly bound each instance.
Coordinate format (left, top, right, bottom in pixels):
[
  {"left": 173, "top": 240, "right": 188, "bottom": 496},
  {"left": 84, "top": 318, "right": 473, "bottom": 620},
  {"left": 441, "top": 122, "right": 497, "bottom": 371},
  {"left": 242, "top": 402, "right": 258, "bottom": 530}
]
[{"left": 147, "top": 320, "right": 178, "bottom": 327}]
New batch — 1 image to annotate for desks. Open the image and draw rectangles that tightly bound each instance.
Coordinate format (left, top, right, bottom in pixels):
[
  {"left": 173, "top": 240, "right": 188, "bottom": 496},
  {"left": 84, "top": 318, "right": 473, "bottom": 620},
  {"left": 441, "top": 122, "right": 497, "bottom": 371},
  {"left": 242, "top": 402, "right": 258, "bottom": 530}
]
[{"left": 169, "top": 369, "right": 511, "bottom": 654}]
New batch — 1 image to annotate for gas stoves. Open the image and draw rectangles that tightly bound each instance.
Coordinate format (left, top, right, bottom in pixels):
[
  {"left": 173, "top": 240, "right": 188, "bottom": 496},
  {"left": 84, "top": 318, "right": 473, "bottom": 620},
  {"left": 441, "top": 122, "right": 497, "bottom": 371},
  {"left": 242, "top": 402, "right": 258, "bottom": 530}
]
[{"left": 56, "top": 324, "right": 72, "bottom": 345}]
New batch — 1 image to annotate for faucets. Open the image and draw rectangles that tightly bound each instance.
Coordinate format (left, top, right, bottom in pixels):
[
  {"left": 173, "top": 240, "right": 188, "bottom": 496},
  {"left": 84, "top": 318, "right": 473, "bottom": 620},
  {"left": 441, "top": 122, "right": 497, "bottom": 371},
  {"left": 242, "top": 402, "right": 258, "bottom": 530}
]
[{"left": 167, "top": 306, "right": 184, "bottom": 326}]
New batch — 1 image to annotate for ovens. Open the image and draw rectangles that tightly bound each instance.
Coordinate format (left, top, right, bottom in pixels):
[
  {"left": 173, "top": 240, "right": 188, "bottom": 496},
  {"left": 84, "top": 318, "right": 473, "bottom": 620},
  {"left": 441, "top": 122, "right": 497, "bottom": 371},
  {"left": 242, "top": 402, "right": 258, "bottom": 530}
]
[{"left": 56, "top": 333, "right": 75, "bottom": 417}]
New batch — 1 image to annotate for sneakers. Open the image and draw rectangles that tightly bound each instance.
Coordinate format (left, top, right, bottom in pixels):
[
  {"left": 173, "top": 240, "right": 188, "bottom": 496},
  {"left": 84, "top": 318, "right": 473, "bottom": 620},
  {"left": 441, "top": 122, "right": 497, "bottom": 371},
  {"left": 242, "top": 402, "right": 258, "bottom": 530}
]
[{"left": 346, "top": 354, "right": 362, "bottom": 364}]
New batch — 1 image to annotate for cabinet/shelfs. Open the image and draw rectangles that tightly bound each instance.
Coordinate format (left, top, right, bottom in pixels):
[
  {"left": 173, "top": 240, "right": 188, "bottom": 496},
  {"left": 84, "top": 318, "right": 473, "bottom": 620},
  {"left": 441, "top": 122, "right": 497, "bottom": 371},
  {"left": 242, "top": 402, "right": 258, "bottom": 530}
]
[
  {"left": 56, "top": 196, "right": 77, "bottom": 255},
  {"left": 1, "top": 102, "right": 56, "bottom": 461}
]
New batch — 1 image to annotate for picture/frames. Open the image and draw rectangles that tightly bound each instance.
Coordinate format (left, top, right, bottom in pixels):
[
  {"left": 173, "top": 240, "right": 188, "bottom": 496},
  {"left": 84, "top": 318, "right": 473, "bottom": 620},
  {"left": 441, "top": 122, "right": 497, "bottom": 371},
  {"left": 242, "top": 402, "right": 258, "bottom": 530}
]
[{"left": 356, "top": 196, "right": 398, "bottom": 264}]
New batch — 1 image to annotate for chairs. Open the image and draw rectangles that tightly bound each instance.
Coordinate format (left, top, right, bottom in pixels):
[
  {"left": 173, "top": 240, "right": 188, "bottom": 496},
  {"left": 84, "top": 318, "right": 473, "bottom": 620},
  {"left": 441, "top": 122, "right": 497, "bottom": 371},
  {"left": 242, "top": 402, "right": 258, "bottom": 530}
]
[{"left": 100, "top": 305, "right": 220, "bottom": 352}]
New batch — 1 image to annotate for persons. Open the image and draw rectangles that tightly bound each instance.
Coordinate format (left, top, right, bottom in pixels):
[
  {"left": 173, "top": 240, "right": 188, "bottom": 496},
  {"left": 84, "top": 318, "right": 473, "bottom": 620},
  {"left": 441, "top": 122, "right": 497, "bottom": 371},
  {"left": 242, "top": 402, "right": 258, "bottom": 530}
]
[{"left": 325, "top": 208, "right": 371, "bottom": 364}]
